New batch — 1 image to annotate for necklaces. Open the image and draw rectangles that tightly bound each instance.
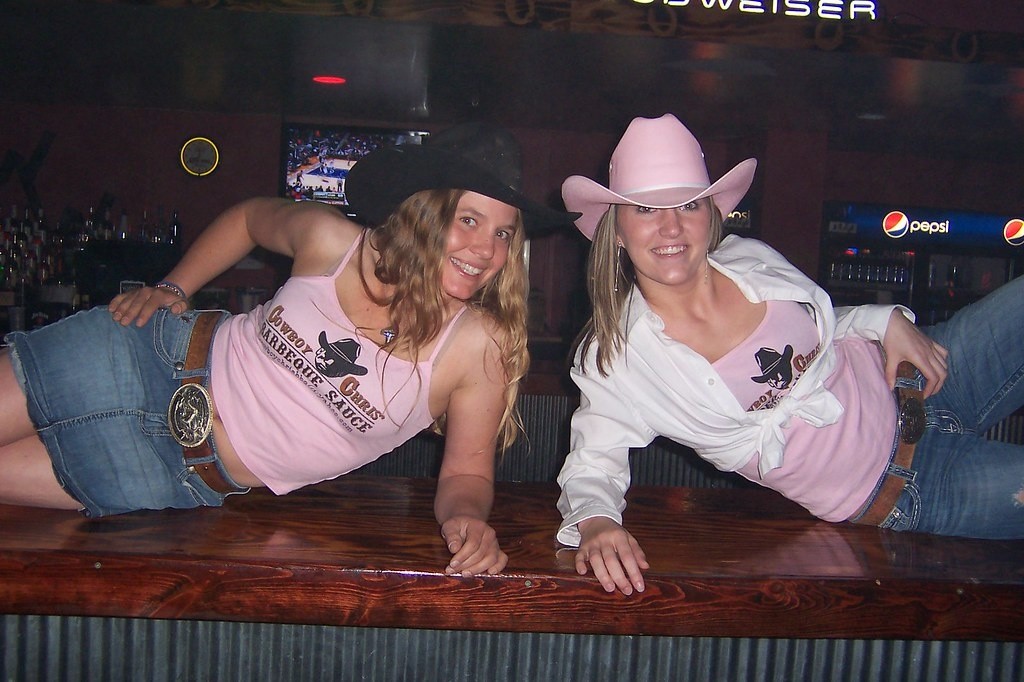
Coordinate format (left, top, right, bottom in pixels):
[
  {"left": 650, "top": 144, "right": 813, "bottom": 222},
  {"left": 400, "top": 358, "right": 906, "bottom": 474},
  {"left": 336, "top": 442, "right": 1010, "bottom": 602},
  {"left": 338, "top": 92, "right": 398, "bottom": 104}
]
[{"left": 380, "top": 326, "right": 396, "bottom": 343}]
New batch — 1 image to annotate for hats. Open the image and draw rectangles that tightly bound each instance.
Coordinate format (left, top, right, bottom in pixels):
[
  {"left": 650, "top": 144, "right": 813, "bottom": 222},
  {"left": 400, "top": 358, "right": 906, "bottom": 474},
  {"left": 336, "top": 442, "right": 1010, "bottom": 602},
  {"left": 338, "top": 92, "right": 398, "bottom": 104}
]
[
  {"left": 561, "top": 113, "right": 757, "bottom": 242},
  {"left": 344, "top": 124, "right": 583, "bottom": 239}
]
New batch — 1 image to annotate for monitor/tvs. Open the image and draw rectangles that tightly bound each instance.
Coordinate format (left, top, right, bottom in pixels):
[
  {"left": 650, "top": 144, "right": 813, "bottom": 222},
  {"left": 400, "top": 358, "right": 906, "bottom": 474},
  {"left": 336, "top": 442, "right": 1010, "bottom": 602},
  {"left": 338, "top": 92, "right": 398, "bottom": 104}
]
[{"left": 279, "top": 122, "right": 431, "bottom": 218}]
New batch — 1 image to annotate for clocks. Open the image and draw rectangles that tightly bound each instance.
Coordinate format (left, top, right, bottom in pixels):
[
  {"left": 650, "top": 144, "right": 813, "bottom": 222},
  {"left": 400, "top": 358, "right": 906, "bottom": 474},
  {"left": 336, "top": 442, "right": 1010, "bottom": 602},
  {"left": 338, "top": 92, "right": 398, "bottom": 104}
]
[{"left": 175, "top": 135, "right": 222, "bottom": 179}]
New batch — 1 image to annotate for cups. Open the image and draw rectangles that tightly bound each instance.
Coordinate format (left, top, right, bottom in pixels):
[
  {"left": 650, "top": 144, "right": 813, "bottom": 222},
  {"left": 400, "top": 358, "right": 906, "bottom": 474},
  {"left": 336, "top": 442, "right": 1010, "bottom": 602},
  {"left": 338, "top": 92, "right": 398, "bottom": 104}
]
[{"left": 8, "top": 306, "right": 26, "bottom": 331}]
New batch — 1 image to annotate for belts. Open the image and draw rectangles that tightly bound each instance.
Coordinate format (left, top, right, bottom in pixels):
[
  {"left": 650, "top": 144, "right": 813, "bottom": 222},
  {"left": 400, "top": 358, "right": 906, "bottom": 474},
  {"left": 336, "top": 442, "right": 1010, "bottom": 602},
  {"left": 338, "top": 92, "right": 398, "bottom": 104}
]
[
  {"left": 854, "top": 361, "right": 926, "bottom": 526},
  {"left": 167, "top": 311, "right": 239, "bottom": 494}
]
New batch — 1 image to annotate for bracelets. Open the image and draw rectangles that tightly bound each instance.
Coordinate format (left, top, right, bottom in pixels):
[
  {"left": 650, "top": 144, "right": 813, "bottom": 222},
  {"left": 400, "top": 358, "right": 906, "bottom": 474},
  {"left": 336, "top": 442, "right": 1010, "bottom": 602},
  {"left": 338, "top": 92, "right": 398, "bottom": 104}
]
[{"left": 154, "top": 281, "right": 186, "bottom": 299}]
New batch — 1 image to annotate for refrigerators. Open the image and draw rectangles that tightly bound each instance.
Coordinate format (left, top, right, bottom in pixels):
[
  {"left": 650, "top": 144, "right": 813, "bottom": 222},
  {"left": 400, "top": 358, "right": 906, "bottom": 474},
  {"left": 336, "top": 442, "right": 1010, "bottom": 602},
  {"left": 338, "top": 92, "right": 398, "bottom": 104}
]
[{"left": 822, "top": 203, "right": 1024, "bottom": 322}]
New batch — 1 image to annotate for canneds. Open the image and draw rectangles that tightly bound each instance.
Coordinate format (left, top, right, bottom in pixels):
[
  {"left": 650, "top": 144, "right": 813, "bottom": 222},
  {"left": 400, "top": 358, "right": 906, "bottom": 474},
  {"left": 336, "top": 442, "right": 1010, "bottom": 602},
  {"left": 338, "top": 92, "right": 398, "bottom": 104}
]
[{"left": 827, "top": 259, "right": 912, "bottom": 287}]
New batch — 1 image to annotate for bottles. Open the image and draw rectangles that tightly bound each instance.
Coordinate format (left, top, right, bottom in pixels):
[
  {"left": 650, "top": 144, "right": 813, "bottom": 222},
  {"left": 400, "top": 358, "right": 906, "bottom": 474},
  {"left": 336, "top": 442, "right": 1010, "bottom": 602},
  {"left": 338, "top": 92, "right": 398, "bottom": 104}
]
[
  {"left": 0, "top": 200, "right": 180, "bottom": 328},
  {"left": 828, "top": 250, "right": 1009, "bottom": 327}
]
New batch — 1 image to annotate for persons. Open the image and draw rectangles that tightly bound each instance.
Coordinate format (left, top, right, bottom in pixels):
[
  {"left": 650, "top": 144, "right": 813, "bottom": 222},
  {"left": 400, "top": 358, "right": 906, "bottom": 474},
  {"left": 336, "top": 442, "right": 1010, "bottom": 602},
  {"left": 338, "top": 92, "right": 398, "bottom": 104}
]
[
  {"left": 286, "top": 130, "right": 422, "bottom": 205},
  {"left": 554, "top": 115, "right": 1024, "bottom": 595},
  {"left": 0, "top": 121, "right": 570, "bottom": 579}
]
